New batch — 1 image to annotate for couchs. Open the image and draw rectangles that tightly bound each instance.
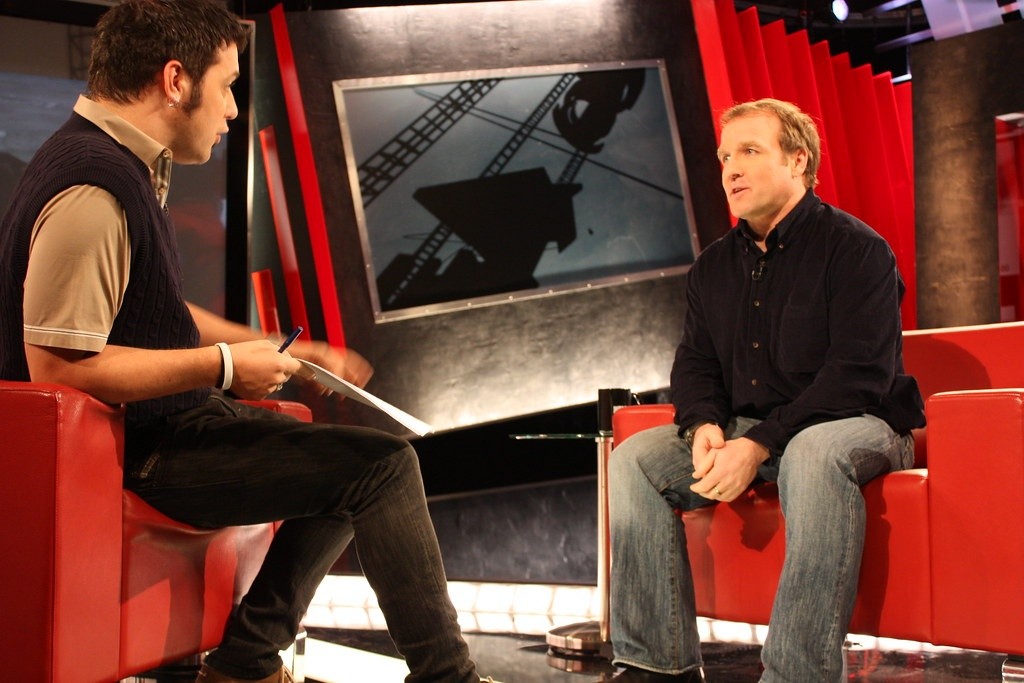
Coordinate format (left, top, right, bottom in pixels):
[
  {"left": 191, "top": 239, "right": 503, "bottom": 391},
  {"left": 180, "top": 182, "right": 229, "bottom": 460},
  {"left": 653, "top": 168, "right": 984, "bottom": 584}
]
[
  {"left": 610, "top": 321, "right": 1024, "bottom": 683},
  {"left": 0, "top": 379, "right": 312, "bottom": 683}
]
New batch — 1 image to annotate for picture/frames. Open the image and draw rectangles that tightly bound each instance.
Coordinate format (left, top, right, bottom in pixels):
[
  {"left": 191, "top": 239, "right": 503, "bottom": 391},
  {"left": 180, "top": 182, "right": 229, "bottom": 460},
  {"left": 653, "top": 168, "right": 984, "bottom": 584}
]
[{"left": 332, "top": 57, "right": 702, "bottom": 327}]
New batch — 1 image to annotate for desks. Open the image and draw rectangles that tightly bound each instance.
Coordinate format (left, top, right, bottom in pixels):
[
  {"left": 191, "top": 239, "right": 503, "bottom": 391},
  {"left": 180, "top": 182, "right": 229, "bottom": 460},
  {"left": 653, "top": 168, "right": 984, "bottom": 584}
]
[{"left": 508, "top": 432, "right": 614, "bottom": 662}]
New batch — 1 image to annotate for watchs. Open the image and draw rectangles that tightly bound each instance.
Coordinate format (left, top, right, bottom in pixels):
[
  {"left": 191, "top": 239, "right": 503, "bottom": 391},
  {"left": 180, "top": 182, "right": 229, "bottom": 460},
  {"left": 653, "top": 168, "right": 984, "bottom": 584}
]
[{"left": 680, "top": 416, "right": 723, "bottom": 456}]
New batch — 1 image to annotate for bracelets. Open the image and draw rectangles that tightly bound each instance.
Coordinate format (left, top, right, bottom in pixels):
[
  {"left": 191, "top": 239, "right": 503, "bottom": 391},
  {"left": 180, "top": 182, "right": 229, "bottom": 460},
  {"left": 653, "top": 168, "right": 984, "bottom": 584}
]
[{"left": 212, "top": 339, "right": 235, "bottom": 392}]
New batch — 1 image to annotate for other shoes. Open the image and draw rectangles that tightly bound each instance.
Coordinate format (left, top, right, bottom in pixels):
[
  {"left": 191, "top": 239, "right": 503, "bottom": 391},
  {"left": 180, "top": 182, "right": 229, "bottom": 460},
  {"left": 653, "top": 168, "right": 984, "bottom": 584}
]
[
  {"left": 194, "top": 663, "right": 295, "bottom": 683},
  {"left": 594, "top": 667, "right": 705, "bottom": 683}
]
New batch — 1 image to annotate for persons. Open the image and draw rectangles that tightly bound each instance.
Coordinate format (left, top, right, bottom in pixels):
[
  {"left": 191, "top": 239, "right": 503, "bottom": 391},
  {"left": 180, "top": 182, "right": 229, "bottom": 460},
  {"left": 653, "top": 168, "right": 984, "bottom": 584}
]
[
  {"left": 594, "top": 98, "right": 932, "bottom": 682},
  {"left": 2, "top": 0, "right": 509, "bottom": 683}
]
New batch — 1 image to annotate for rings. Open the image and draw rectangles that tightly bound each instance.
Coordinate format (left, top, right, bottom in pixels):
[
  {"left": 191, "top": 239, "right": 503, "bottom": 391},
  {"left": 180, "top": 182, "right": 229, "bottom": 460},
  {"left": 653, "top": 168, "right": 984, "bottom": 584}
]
[
  {"left": 713, "top": 487, "right": 721, "bottom": 495},
  {"left": 276, "top": 380, "right": 284, "bottom": 393}
]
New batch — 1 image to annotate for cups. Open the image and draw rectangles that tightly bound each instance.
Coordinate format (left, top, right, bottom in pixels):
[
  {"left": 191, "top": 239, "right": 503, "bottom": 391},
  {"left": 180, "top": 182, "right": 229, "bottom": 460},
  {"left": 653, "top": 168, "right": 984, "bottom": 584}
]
[{"left": 597, "top": 388, "right": 642, "bottom": 437}]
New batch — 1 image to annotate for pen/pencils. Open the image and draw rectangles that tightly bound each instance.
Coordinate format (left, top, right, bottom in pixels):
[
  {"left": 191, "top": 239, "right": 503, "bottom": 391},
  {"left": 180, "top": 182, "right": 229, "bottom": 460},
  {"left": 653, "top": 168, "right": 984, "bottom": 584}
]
[{"left": 277, "top": 326, "right": 303, "bottom": 353}]
